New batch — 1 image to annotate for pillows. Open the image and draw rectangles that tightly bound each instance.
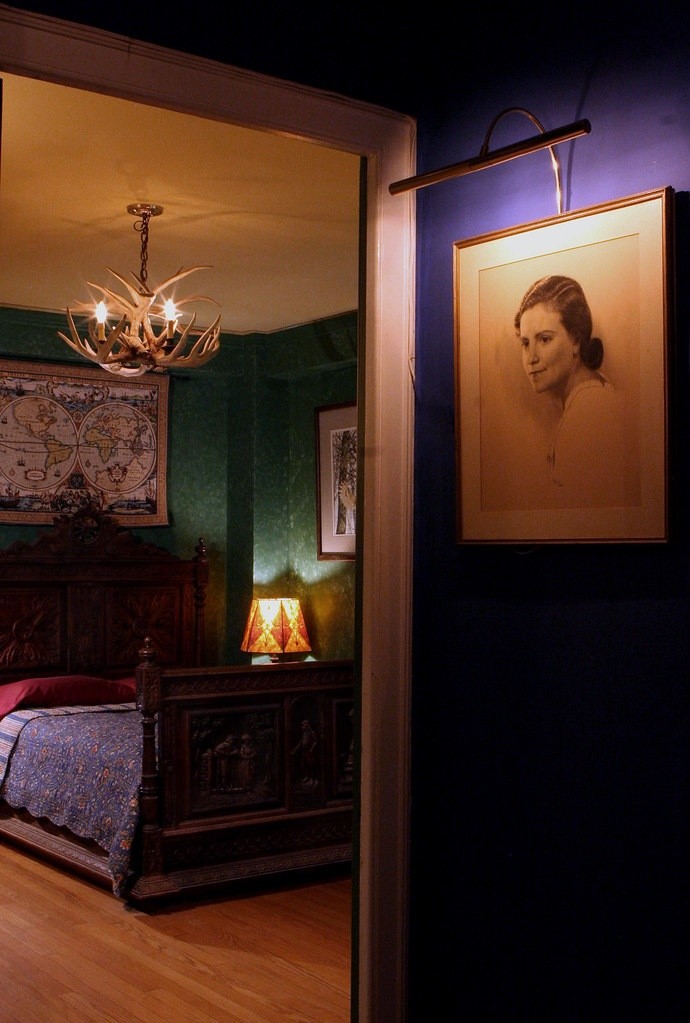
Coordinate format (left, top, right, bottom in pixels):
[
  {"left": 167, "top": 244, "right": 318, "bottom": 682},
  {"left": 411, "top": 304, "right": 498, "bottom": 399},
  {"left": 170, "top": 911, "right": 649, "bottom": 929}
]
[
  {"left": 0, "top": 674, "right": 137, "bottom": 720},
  {"left": 110, "top": 675, "right": 137, "bottom": 698}
]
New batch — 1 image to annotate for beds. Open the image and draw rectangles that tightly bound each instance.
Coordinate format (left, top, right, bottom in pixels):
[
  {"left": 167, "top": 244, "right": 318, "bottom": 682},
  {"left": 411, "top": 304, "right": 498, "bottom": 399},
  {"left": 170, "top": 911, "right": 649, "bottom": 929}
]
[{"left": 0, "top": 502, "right": 355, "bottom": 900}]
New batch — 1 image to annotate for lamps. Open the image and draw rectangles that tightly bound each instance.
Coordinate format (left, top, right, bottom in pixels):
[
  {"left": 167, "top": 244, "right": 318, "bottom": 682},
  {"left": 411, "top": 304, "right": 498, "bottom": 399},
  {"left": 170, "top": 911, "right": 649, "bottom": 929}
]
[
  {"left": 57, "top": 203, "right": 223, "bottom": 378},
  {"left": 240, "top": 597, "right": 318, "bottom": 665}
]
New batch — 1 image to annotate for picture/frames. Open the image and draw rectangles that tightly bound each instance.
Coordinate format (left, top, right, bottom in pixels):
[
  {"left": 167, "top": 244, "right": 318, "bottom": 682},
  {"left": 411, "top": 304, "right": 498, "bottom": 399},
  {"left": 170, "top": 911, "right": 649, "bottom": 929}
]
[
  {"left": 451, "top": 185, "right": 678, "bottom": 545},
  {"left": 315, "top": 405, "right": 358, "bottom": 562}
]
